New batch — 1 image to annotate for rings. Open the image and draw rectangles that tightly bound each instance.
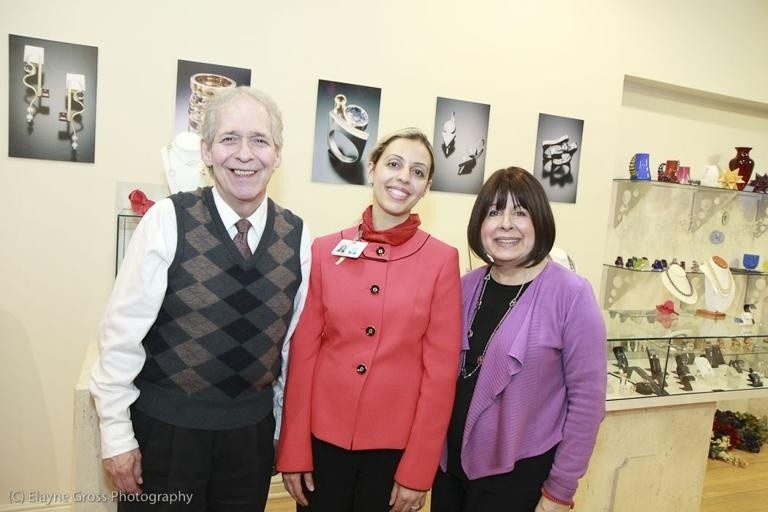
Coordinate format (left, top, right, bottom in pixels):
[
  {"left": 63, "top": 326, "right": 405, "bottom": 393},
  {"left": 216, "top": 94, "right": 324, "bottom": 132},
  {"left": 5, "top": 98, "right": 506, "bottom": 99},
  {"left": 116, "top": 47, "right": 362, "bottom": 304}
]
[{"left": 412, "top": 505, "right": 421, "bottom": 509}]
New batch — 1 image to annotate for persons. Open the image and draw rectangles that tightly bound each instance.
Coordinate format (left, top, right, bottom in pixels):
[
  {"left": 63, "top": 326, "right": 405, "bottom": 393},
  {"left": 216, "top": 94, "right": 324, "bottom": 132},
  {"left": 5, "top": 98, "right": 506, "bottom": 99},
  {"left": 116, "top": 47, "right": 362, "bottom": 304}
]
[
  {"left": 275, "top": 127, "right": 463, "bottom": 512},
  {"left": 90, "top": 85, "right": 312, "bottom": 512},
  {"left": 431, "top": 167, "right": 608, "bottom": 512}
]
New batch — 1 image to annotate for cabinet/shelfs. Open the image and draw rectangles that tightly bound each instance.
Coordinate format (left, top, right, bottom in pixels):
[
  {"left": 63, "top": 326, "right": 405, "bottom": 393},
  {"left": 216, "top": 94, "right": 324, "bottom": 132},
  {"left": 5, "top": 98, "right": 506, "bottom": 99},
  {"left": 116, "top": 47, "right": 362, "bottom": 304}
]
[{"left": 603, "top": 178, "right": 768, "bottom": 402}]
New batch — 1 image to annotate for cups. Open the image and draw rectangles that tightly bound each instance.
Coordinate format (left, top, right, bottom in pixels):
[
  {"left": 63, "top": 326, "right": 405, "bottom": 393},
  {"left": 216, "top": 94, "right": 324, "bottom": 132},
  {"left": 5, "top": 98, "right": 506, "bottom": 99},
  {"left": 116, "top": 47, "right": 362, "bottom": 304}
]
[
  {"left": 188, "top": 73, "right": 238, "bottom": 133},
  {"left": 743, "top": 253, "right": 760, "bottom": 270}
]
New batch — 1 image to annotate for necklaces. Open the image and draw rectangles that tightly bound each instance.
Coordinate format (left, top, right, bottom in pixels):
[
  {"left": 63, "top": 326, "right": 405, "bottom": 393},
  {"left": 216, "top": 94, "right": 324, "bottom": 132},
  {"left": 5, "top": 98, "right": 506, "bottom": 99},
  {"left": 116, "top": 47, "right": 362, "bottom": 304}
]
[
  {"left": 708, "top": 262, "right": 733, "bottom": 294},
  {"left": 666, "top": 266, "right": 694, "bottom": 296},
  {"left": 458, "top": 261, "right": 542, "bottom": 380}
]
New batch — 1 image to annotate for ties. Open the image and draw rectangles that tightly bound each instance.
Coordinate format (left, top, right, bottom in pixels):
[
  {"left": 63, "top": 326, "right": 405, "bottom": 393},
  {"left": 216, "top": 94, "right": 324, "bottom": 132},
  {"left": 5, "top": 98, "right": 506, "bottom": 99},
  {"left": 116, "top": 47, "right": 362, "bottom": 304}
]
[{"left": 231, "top": 219, "right": 252, "bottom": 260}]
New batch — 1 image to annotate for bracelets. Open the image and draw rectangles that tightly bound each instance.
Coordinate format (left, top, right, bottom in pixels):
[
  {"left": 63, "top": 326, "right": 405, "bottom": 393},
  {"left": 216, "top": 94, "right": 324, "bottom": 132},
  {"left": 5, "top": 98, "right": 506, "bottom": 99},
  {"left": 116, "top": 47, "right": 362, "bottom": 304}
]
[{"left": 540, "top": 486, "right": 575, "bottom": 509}]
